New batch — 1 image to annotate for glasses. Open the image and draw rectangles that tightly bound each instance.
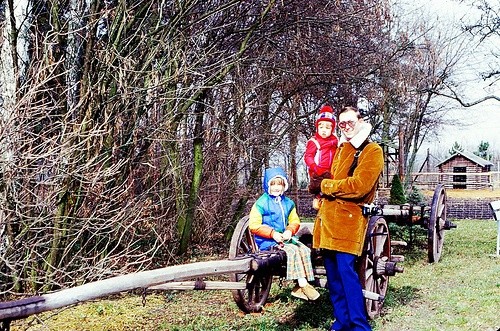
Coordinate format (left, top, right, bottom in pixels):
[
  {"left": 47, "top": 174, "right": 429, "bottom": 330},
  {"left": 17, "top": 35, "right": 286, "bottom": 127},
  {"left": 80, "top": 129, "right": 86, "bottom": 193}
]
[{"left": 338, "top": 120, "right": 359, "bottom": 130}]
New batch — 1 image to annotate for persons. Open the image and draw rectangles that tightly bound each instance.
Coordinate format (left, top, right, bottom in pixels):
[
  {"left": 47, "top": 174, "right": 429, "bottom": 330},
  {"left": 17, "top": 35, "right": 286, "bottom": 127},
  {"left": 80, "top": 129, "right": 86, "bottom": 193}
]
[
  {"left": 304, "top": 106, "right": 339, "bottom": 210},
  {"left": 249, "top": 167, "right": 320, "bottom": 300},
  {"left": 309, "top": 107, "right": 384, "bottom": 331}
]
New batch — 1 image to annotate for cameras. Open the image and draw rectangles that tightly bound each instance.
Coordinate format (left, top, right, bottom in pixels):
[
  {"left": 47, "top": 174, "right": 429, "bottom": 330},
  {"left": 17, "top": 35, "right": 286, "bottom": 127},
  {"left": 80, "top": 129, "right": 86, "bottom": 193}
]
[{"left": 362, "top": 205, "right": 381, "bottom": 215}]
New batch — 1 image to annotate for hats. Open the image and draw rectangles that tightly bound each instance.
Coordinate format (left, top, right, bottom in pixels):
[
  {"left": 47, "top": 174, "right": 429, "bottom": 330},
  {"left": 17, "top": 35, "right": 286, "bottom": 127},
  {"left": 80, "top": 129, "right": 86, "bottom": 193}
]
[{"left": 316, "top": 106, "right": 336, "bottom": 135}]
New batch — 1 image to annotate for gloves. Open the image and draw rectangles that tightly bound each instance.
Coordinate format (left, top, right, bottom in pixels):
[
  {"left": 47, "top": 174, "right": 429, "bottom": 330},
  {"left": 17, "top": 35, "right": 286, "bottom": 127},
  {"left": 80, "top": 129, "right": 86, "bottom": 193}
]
[{"left": 307, "top": 163, "right": 332, "bottom": 195}]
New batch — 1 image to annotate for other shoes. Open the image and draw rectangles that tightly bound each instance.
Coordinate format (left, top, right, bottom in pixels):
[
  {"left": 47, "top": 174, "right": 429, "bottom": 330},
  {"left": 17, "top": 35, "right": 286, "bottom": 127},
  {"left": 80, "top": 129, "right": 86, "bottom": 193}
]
[
  {"left": 290, "top": 284, "right": 309, "bottom": 301},
  {"left": 299, "top": 282, "right": 321, "bottom": 301}
]
